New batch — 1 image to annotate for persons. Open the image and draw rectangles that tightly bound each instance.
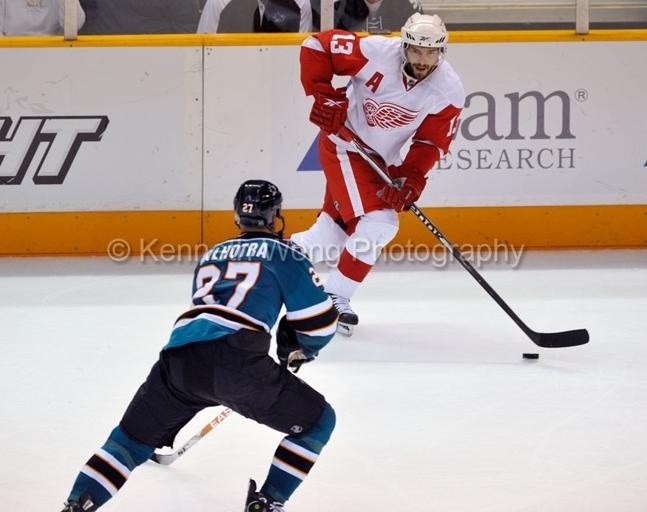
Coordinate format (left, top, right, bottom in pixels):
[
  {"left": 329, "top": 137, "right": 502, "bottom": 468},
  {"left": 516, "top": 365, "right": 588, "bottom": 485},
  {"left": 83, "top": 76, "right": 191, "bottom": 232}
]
[
  {"left": 194, "top": 1, "right": 314, "bottom": 37},
  {"left": 333, "top": 0, "right": 421, "bottom": 33},
  {"left": 286, "top": 11, "right": 463, "bottom": 327},
  {"left": 60, "top": 179, "right": 337, "bottom": 511},
  {"left": 0, "top": 0, "right": 85, "bottom": 37}
]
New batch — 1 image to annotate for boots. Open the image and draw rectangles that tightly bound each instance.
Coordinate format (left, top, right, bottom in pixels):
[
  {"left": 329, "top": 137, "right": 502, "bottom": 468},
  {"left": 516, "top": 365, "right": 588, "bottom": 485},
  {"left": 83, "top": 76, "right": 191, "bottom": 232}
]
[
  {"left": 244, "top": 477, "right": 285, "bottom": 512},
  {"left": 320, "top": 292, "right": 359, "bottom": 337},
  {"left": 62, "top": 492, "right": 98, "bottom": 512}
]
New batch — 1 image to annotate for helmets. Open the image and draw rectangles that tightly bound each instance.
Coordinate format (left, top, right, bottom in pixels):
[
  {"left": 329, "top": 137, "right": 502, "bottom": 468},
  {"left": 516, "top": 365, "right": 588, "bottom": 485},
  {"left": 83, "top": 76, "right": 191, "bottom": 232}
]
[
  {"left": 400, "top": 12, "right": 449, "bottom": 55},
  {"left": 253, "top": 0, "right": 300, "bottom": 32},
  {"left": 233, "top": 180, "right": 283, "bottom": 228}
]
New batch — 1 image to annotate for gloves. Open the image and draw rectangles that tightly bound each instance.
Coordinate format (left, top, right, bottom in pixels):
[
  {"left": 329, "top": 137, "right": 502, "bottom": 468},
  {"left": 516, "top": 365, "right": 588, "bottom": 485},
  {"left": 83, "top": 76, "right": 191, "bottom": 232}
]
[
  {"left": 308, "top": 87, "right": 349, "bottom": 133},
  {"left": 276, "top": 314, "right": 308, "bottom": 366},
  {"left": 382, "top": 163, "right": 428, "bottom": 210}
]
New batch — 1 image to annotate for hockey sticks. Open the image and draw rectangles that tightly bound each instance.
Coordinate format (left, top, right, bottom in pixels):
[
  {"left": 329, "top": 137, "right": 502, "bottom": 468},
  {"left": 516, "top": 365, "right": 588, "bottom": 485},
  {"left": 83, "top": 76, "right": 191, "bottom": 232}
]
[
  {"left": 339, "top": 125, "right": 589, "bottom": 348},
  {"left": 149, "top": 408, "right": 233, "bottom": 466}
]
